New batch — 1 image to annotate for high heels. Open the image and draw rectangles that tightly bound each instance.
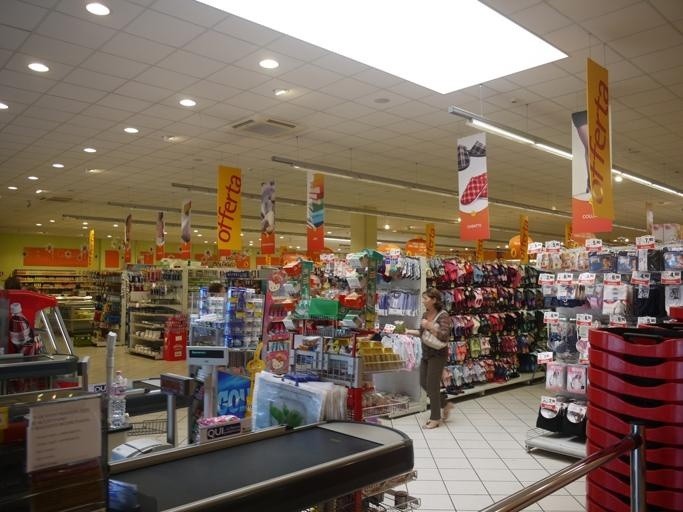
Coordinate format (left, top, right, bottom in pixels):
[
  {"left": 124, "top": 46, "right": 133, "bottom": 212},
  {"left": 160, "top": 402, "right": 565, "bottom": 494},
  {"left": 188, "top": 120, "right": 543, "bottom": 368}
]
[
  {"left": 422, "top": 419, "right": 440, "bottom": 429},
  {"left": 442, "top": 402, "right": 455, "bottom": 420}
]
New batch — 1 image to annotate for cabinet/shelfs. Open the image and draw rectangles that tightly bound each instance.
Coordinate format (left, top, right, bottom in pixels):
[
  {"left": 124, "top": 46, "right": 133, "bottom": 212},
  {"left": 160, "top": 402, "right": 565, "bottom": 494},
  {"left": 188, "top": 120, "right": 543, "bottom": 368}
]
[{"left": 13, "top": 269, "right": 290, "bottom": 376}]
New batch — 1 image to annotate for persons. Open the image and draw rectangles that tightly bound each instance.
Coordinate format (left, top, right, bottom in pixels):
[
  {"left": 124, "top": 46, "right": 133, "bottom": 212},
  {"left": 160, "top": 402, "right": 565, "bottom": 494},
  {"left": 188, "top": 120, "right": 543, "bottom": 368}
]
[
  {"left": 209, "top": 283, "right": 225, "bottom": 297},
  {"left": 404, "top": 289, "right": 456, "bottom": 429}
]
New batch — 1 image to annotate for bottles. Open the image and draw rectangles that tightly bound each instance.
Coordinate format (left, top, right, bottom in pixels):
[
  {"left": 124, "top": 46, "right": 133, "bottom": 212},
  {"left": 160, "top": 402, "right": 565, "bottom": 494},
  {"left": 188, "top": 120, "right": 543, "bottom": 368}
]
[
  {"left": 108, "top": 371, "right": 127, "bottom": 428},
  {"left": 8, "top": 303, "right": 34, "bottom": 353}
]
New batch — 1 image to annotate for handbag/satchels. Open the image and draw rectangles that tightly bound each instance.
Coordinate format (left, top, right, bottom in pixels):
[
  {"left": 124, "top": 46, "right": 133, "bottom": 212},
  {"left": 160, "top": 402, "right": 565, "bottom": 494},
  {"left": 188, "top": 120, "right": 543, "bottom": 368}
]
[
  {"left": 422, "top": 329, "right": 447, "bottom": 351},
  {"left": 251, "top": 370, "right": 350, "bottom": 443}
]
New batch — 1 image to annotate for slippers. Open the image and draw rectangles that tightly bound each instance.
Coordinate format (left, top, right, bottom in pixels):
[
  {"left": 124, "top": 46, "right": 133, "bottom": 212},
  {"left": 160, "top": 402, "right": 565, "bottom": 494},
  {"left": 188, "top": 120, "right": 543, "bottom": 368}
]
[
  {"left": 430, "top": 260, "right": 440, "bottom": 278},
  {"left": 440, "top": 260, "right": 539, "bottom": 389}
]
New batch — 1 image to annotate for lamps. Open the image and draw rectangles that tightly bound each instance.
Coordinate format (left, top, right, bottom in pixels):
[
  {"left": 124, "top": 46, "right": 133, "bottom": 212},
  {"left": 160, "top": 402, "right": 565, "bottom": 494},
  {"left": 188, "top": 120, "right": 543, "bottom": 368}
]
[{"left": 449, "top": 105, "right": 682, "bottom": 198}]
